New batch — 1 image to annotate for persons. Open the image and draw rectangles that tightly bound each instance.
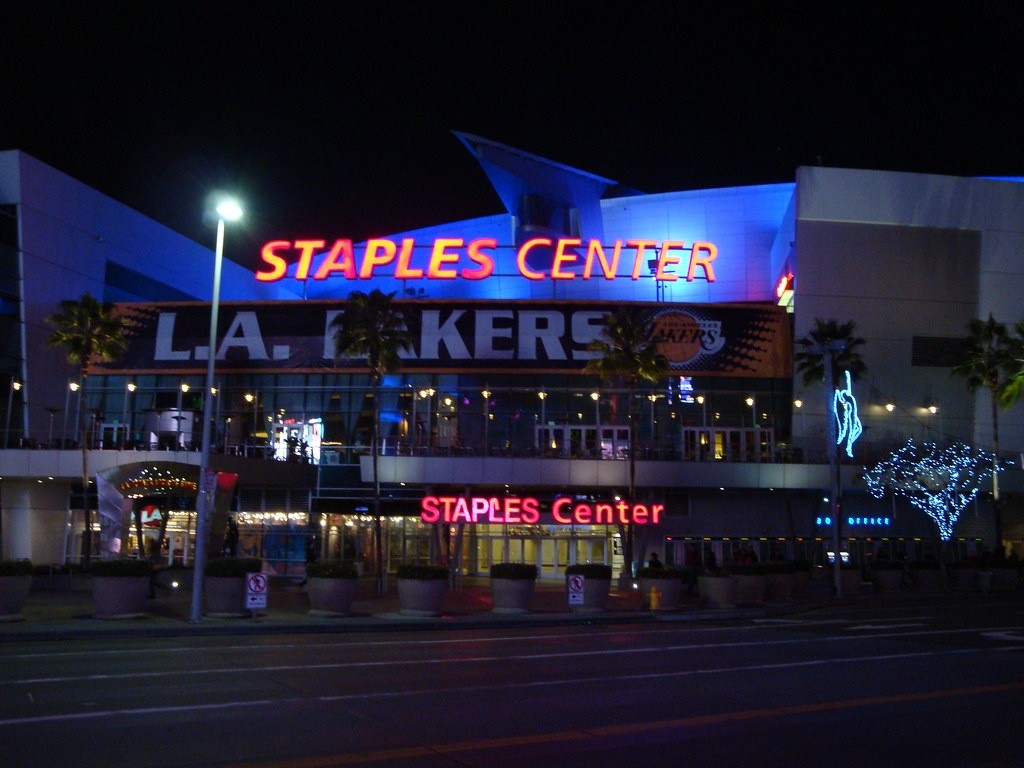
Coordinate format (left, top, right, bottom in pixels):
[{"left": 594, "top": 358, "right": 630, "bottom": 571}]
[
  {"left": 648, "top": 553, "right": 664, "bottom": 569},
  {"left": 733, "top": 542, "right": 758, "bottom": 568}
]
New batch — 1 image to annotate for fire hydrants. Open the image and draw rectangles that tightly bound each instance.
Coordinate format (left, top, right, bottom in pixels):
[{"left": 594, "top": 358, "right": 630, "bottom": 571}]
[{"left": 647, "top": 586, "right": 663, "bottom": 612}]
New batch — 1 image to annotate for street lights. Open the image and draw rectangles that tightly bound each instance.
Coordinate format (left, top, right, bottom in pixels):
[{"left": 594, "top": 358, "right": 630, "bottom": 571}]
[{"left": 189, "top": 200, "right": 244, "bottom": 624}]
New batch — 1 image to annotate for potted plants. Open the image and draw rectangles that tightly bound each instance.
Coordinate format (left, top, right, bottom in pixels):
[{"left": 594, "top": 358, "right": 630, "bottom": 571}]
[
  {"left": 0, "top": 559, "right": 35, "bottom": 624},
  {"left": 489, "top": 563, "right": 537, "bottom": 614},
  {"left": 638, "top": 567, "right": 681, "bottom": 607},
  {"left": 788, "top": 556, "right": 1020, "bottom": 598},
  {"left": 564, "top": 564, "right": 612, "bottom": 612},
  {"left": 304, "top": 562, "right": 359, "bottom": 617},
  {"left": 739, "top": 561, "right": 777, "bottom": 604},
  {"left": 395, "top": 566, "right": 451, "bottom": 615},
  {"left": 697, "top": 568, "right": 738, "bottom": 610},
  {"left": 88, "top": 560, "right": 153, "bottom": 618},
  {"left": 202, "top": 558, "right": 263, "bottom": 618}
]
